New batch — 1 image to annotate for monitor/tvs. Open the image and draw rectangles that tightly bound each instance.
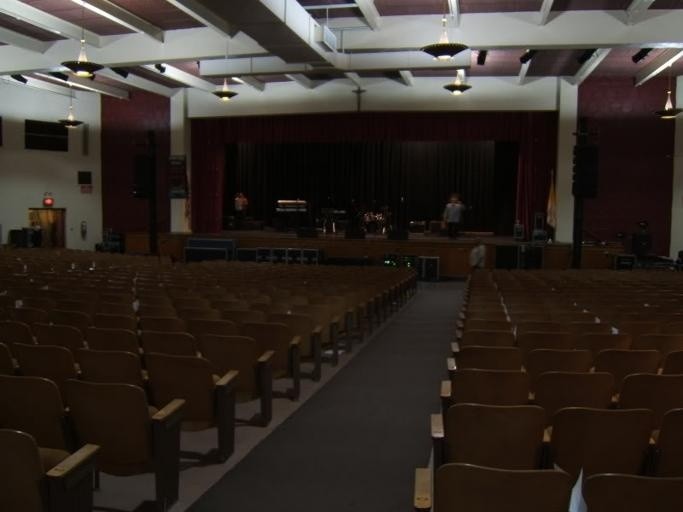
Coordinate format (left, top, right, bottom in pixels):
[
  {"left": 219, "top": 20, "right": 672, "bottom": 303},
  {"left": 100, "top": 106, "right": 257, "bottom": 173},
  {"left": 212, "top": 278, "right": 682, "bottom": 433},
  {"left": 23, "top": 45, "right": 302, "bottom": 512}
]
[{"left": 24, "top": 120, "right": 69, "bottom": 151}]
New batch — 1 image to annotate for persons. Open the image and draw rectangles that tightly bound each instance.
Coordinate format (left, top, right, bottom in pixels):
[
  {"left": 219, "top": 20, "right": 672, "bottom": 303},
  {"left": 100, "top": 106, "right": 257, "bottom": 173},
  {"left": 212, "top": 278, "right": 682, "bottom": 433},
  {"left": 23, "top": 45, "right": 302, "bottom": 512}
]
[
  {"left": 443, "top": 196, "right": 466, "bottom": 239},
  {"left": 469, "top": 237, "right": 486, "bottom": 274},
  {"left": 234, "top": 192, "right": 248, "bottom": 230},
  {"left": 31, "top": 221, "right": 42, "bottom": 248}
]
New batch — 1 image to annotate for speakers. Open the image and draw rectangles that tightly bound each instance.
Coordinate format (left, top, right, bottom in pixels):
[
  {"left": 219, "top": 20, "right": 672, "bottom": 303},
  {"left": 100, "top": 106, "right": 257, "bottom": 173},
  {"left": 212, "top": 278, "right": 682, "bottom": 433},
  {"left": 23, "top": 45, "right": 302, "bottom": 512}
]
[
  {"left": 572, "top": 144, "right": 598, "bottom": 199},
  {"left": 134, "top": 153, "right": 152, "bottom": 200}
]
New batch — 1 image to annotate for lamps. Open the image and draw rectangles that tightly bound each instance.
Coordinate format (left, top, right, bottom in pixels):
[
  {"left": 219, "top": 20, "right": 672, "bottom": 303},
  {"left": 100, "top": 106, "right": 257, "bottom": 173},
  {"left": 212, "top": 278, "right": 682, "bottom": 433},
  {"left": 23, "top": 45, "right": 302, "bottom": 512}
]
[
  {"left": 212, "top": 76, "right": 238, "bottom": 102},
  {"left": 654, "top": 64, "right": 682, "bottom": 121},
  {"left": 58, "top": 7, "right": 103, "bottom": 77},
  {"left": 417, "top": 1, "right": 468, "bottom": 60},
  {"left": 57, "top": 88, "right": 85, "bottom": 129},
  {"left": 444, "top": 68, "right": 472, "bottom": 96}
]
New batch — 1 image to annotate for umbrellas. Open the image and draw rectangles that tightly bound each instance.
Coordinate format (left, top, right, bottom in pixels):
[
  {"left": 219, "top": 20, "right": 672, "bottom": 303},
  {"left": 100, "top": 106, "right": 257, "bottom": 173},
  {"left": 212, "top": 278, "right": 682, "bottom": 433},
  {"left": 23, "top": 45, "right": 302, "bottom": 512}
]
[{"left": 546, "top": 169, "right": 556, "bottom": 228}]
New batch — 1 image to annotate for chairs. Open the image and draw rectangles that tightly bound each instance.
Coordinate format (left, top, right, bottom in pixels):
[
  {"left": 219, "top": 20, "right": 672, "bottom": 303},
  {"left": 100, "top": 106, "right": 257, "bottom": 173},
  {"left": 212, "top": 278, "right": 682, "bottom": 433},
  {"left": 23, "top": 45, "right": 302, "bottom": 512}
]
[
  {"left": 1, "top": 245, "right": 419, "bottom": 510},
  {"left": 413, "top": 264, "right": 683, "bottom": 510}
]
